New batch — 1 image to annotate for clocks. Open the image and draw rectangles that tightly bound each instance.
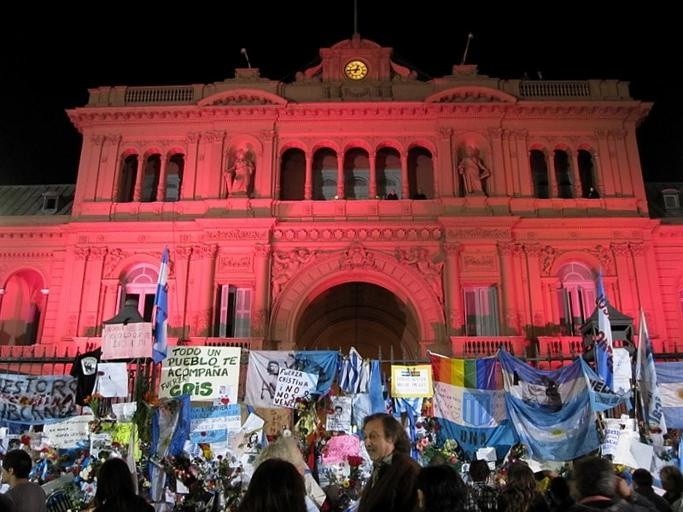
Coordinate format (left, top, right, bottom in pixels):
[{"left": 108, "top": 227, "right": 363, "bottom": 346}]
[{"left": 341, "top": 56, "right": 371, "bottom": 84}]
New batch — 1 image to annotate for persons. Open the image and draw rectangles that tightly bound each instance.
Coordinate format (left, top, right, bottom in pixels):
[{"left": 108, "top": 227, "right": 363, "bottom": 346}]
[
  {"left": 84, "top": 457, "right": 158, "bottom": 511},
  {"left": 224, "top": 150, "right": 254, "bottom": 193},
  {"left": 591, "top": 246, "right": 612, "bottom": 270},
  {"left": 542, "top": 246, "right": 558, "bottom": 273},
  {"left": 0, "top": 449, "right": 45, "bottom": 512},
  {"left": 459, "top": 145, "right": 487, "bottom": 191},
  {"left": 238, "top": 416, "right": 683, "bottom": 512},
  {"left": 401, "top": 249, "right": 443, "bottom": 301},
  {"left": 271, "top": 249, "right": 318, "bottom": 298}
]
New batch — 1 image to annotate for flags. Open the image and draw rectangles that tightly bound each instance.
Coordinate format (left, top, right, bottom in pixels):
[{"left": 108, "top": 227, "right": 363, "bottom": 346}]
[
  {"left": 246, "top": 276, "right": 682, "bottom": 459},
  {"left": 153, "top": 248, "right": 169, "bottom": 362}
]
